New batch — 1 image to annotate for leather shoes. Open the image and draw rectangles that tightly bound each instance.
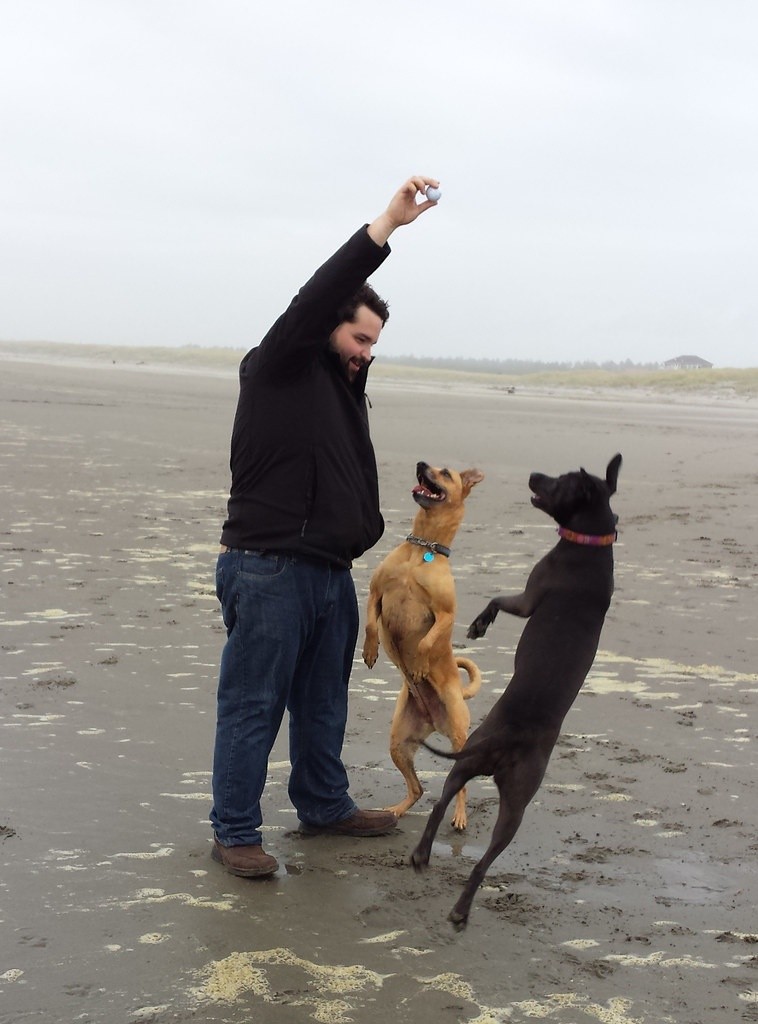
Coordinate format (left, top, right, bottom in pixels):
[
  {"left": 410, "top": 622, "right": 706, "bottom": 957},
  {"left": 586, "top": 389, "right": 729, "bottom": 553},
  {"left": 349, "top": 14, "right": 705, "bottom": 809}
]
[
  {"left": 209, "top": 840, "right": 279, "bottom": 879},
  {"left": 298, "top": 808, "right": 398, "bottom": 837}
]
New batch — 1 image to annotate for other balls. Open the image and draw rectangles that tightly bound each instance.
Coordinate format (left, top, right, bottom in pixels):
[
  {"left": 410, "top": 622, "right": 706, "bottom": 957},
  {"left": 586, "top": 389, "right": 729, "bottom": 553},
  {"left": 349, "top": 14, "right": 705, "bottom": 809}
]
[{"left": 426, "top": 185, "right": 441, "bottom": 202}]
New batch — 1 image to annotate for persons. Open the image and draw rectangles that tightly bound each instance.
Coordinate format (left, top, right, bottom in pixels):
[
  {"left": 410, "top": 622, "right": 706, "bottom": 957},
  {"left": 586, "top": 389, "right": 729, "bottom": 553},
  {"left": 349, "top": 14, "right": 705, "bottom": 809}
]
[{"left": 208, "top": 173, "right": 444, "bottom": 877}]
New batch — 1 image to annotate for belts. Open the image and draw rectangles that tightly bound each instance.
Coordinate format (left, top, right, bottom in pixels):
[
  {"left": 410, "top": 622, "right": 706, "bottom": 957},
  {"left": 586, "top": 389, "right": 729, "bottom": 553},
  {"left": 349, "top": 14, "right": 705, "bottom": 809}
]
[{"left": 226, "top": 547, "right": 296, "bottom": 561}]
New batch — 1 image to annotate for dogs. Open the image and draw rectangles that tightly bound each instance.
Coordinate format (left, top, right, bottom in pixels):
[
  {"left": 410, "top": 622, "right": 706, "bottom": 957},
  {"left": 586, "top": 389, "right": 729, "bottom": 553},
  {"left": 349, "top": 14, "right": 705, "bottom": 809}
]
[
  {"left": 408, "top": 449, "right": 623, "bottom": 931},
  {"left": 362, "top": 460, "right": 483, "bottom": 833}
]
